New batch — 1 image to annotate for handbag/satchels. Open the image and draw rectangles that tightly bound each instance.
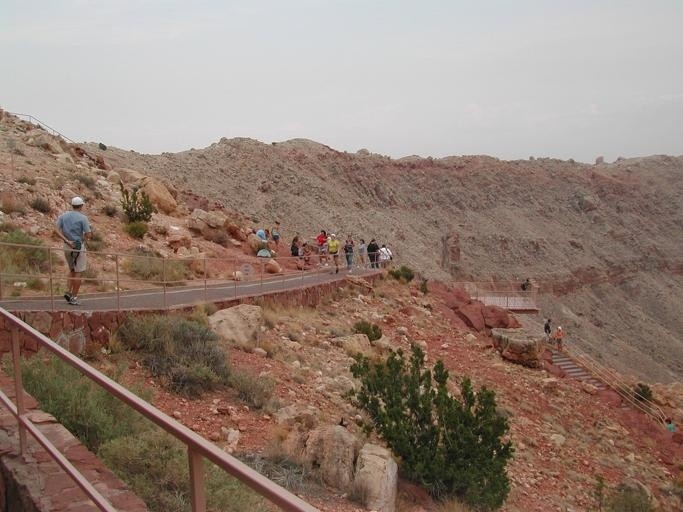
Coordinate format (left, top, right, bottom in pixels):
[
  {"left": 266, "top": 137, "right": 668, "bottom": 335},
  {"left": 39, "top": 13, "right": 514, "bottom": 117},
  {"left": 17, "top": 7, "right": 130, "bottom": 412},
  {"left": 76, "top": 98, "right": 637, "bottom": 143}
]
[
  {"left": 272, "top": 232, "right": 280, "bottom": 240},
  {"left": 71, "top": 241, "right": 81, "bottom": 251}
]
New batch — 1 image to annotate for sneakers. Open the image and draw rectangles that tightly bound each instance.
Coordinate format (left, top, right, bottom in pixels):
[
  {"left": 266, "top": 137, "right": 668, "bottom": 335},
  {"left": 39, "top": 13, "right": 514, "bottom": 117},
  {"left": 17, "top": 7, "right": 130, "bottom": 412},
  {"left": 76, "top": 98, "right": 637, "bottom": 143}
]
[
  {"left": 316, "top": 261, "right": 329, "bottom": 267},
  {"left": 357, "top": 265, "right": 390, "bottom": 271},
  {"left": 328, "top": 268, "right": 352, "bottom": 275},
  {"left": 64, "top": 291, "right": 80, "bottom": 305}
]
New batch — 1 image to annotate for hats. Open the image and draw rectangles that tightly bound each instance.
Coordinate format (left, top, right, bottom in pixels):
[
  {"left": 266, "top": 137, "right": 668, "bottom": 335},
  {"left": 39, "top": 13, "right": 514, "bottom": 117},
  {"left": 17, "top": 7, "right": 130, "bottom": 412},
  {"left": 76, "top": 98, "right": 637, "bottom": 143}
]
[
  {"left": 71, "top": 196, "right": 85, "bottom": 206},
  {"left": 331, "top": 233, "right": 336, "bottom": 237}
]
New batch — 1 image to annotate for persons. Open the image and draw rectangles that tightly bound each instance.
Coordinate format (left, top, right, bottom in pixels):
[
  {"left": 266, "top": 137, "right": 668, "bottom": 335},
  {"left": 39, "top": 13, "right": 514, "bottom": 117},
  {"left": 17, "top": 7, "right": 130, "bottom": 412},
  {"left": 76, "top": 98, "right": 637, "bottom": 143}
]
[
  {"left": 522, "top": 279, "right": 530, "bottom": 291},
  {"left": 544, "top": 319, "right": 552, "bottom": 342},
  {"left": 257, "top": 220, "right": 392, "bottom": 274},
  {"left": 665, "top": 417, "right": 675, "bottom": 433},
  {"left": 556, "top": 326, "right": 563, "bottom": 352},
  {"left": 54, "top": 197, "right": 93, "bottom": 307}
]
